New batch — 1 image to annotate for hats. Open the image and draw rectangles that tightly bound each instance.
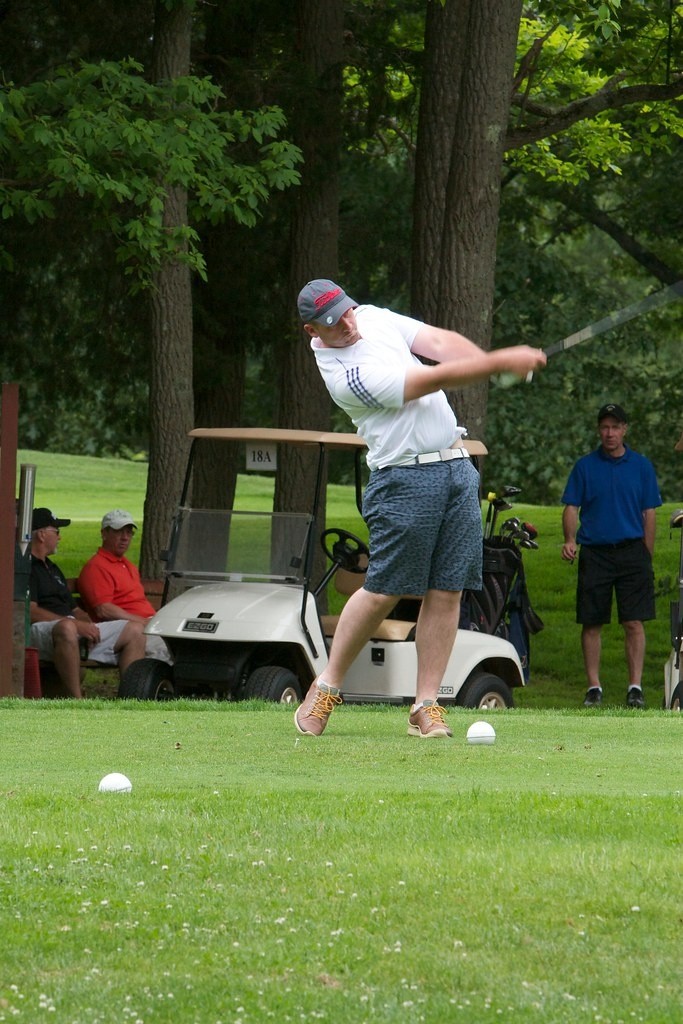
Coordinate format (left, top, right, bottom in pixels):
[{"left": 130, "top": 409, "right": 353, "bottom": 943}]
[
  {"left": 296, "top": 279, "right": 358, "bottom": 327},
  {"left": 597, "top": 403, "right": 628, "bottom": 428},
  {"left": 101, "top": 510, "right": 137, "bottom": 533},
  {"left": 31, "top": 507, "right": 70, "bottom": 532}
]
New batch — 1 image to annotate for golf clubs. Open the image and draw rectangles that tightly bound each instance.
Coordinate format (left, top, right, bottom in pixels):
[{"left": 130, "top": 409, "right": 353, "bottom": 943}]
[
  {"left": 669, "top": 508, "right": 683, "bottom": 671},
  {"left": 484, "top": 485, "right": 539, "bottom": 551},
  {"left": 498, "top": 281, "right": 683, "bottom": 390}
]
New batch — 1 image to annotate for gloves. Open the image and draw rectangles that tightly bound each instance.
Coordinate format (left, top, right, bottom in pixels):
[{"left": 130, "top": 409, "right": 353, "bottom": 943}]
[{"left": 490, "top": 368, "right": 534, "bottom": 389}]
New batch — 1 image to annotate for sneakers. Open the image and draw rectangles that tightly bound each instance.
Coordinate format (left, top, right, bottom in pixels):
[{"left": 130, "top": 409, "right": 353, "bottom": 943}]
[
  {"left": 293, "top": 675, "right": 344, "bottom": 736},
  {"left": 406, "top": 700, "right": 454, "bottom": 739},
  {"left": 583, "top": 686, "right": 604, "bottom": 709},
  {"left": 627, "top": 686, "right": 646, "bottom": 710}
]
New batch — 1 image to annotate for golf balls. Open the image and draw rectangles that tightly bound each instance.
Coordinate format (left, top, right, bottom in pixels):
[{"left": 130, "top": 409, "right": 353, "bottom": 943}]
[
  {"left": 467, "top": 720, "right": 497, "bottom": 746},
  {"left": 100, "top": 772, "right": 132, "bottom": 794}
]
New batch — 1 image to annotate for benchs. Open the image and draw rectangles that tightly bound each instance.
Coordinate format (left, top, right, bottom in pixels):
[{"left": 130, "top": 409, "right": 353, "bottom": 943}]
[
  {"left": 12, "top": 579, "right": 178, "bottom": 701},
  {"left": 319, "top": 554, "right": 424, "bottom": 641}
]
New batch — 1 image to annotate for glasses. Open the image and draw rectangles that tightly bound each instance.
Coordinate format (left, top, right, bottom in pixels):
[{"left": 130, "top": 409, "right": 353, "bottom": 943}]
[{"left": 46, "top": 529, "right": 60, "bottom": 536}]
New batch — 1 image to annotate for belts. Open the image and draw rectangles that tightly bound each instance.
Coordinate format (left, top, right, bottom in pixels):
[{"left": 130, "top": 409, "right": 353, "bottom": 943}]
[{"left": 394, "top": 448, "right": 471, "bottom": 467}]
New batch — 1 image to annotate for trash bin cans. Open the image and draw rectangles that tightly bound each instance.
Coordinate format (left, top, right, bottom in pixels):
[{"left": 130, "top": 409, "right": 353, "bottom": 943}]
[{"left": 24, "top": 648, "right": 41, "bottom": 698}]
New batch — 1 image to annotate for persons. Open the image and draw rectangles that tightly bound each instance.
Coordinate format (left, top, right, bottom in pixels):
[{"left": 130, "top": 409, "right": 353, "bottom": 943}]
[
  {"left": 560, "top": 406, "right": 663, "bottom": 707},
  {"left": 290, "top": 276, "right": 551, "bottom": 738},
  {"left": 24, "top": 508, "right": 174, "bottom": 700}
]
